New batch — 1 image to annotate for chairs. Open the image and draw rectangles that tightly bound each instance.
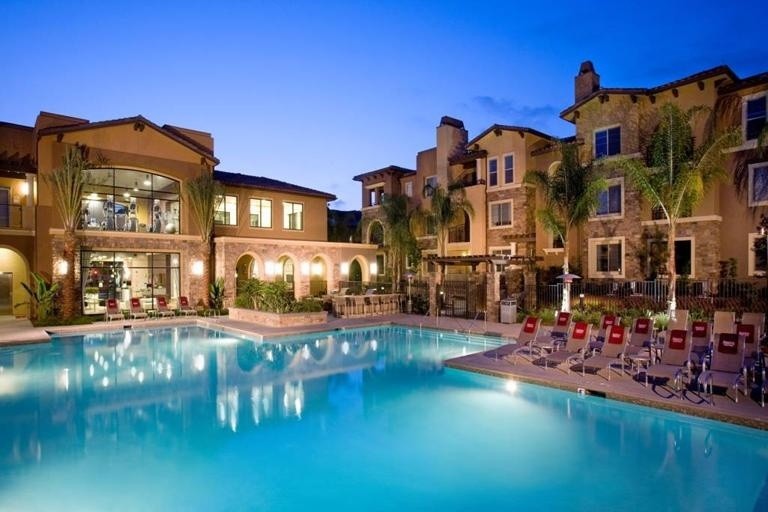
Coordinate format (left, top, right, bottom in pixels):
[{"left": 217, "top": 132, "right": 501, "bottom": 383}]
[
  {"left": 482, "top": 306, "right": 767, "bottom": 408},
  {"left": 103, "top": 296, "right": 198, "bottom": 325}
]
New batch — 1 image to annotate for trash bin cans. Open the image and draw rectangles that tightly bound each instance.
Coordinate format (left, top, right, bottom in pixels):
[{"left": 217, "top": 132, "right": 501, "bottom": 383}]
[{"left": 500, "top": 299, "right": 517, "bottom": 324}]
